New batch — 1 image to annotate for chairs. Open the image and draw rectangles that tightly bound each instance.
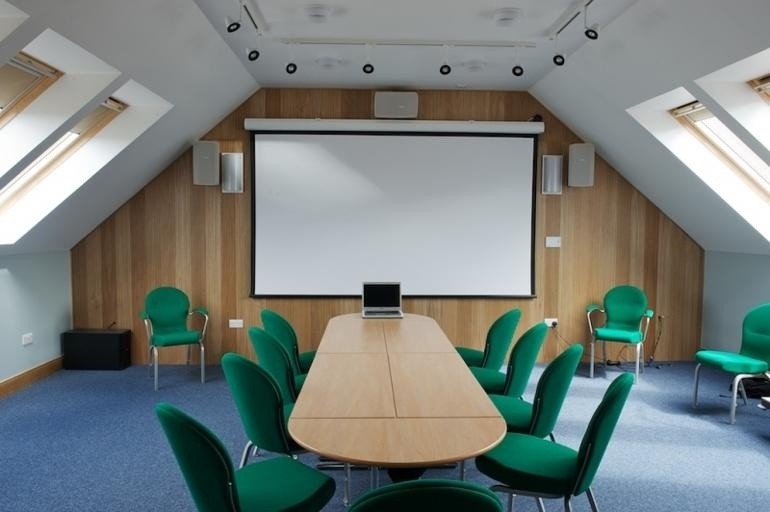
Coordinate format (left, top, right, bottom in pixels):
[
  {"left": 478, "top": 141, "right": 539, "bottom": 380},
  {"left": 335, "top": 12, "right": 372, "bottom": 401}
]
[
  {"left": 478, "top": 370, "right": 635, "bottom": 510},
  {"left": 260, "top": 303, "right": 315, "bottom": 374},
  {"left": 453, "top": 309, "right": 522, "bottom": 370},
  {"left": 693, "top": 303, "right": 769, "bottom": 423},
  {"left": 155, "top": 402, "right": 336, "bottom": 511},
  {"left": 140, "top": 285, "right": 208, "bottom": 393},
  {"left": 220, "top": 352, "right": 314, "bottom": 469},
  {"left": 348, "top": 479, "right": 501, "bottom": 510},
  {"left": 248, "top": 327, "right": 302, "bottom": 424},
  {"left": 465, "top": 325, "right": 548, "bottom": 400},
  {"left": 488, "top": 344, "right": 584, "bottom": 443},
  {"left": 585, "top": 283, "right": 653, "bottom": 383}
]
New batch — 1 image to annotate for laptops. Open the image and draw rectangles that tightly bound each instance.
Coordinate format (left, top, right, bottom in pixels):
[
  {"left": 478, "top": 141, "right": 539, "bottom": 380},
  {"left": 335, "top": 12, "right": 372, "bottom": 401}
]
[{"left": 362, "top": 281, "right": 404, "bottom": 319}]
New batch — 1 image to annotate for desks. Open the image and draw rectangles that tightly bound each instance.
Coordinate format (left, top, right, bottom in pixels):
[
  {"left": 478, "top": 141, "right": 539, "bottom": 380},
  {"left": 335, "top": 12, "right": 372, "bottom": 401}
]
[{"left": 288, "top": 313, "right": 508, "bottom": 508}]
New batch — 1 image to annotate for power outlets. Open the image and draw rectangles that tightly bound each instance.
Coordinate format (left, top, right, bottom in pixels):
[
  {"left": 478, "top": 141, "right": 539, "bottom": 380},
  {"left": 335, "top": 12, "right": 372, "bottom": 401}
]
[{"left": 544, "top": 319, "right": 559, "bottom": 328}]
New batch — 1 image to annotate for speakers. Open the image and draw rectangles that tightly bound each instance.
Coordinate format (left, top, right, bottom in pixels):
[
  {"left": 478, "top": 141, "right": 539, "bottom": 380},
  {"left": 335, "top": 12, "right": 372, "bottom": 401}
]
[
  {"left": 192, "top": 140, "right": 220, "bottom": 186},
  {"left": 374, "top": 91, "right": 419, "bottom": 119},
  {"left": 568, "top": 143, "right": 596, "bottom": 188},
  {"left": 62, "top": 329, "right": 132, "bottom": 371},
  {"left": 541, "top": 154, "right": 564, "bottom": 195},
  {"left": 220, "top": 152, "right": 244, "bottom": 194}
]
